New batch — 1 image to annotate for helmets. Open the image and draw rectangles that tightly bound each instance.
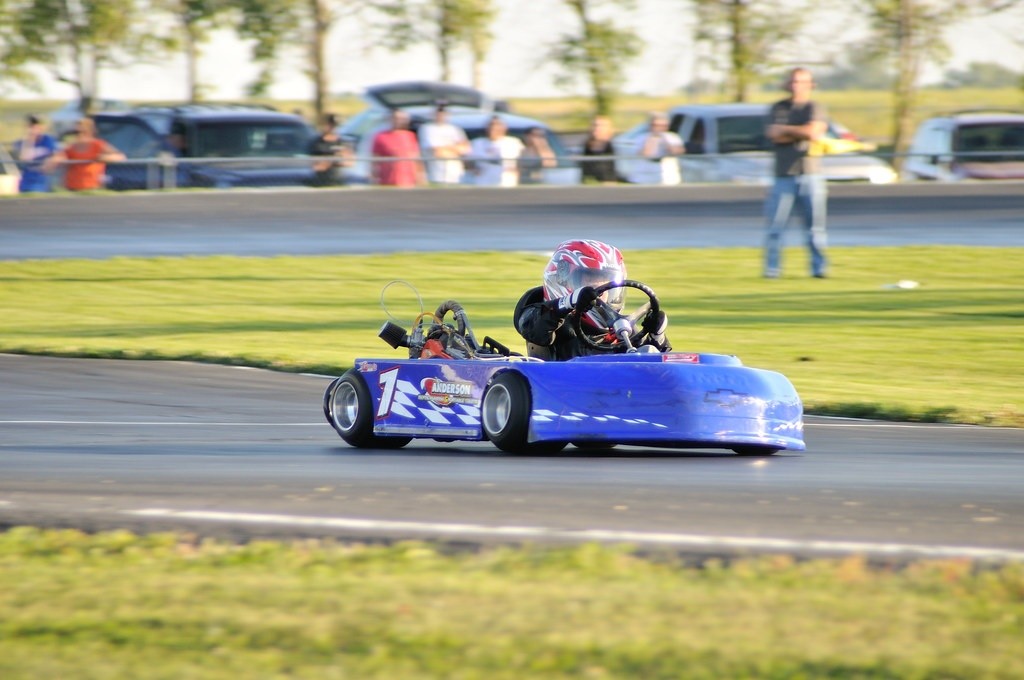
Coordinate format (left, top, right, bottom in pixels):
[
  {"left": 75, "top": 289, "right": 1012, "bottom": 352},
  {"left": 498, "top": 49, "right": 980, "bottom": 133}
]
[{"left": 542, "top": 239, "right": 627, "bottom": 333}]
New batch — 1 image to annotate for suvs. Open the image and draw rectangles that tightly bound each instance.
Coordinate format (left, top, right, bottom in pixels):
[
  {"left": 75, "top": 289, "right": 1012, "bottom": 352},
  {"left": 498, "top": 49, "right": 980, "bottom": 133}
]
[{"left": 84, "top": 102, "right": 373, "bottom": 190}]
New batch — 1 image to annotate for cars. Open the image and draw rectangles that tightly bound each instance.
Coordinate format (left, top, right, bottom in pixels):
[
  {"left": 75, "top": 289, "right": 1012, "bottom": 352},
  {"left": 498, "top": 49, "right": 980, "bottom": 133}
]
[
  {"left": 333, "top": 80, "right": 584, "bottom": 185},
  {"left": 901, "top": 108, "right": 1024, "bottom": 183}
]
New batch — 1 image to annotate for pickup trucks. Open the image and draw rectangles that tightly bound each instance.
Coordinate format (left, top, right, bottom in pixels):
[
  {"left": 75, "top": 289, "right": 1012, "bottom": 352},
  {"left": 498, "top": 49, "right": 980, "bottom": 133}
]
[{"left": 613, "top": 101, "right": 900, "bottom": 187}]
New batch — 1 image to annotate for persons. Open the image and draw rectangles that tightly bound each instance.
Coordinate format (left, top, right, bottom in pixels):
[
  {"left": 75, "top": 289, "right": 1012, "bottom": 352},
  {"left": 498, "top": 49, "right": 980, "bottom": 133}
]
[
  {"left": 631, "top": 114, "right": 685, "bottom": 185},
  {"left": 9, "top": 113, "right": 127, "bottom": 192},
  {"left": 143, "top": 125, "right": 191, "bottom": 188},
  {"left": 576, "top": 115, "right": 618, "bottom": 184},
  {"left": 372, "top": 106, "right": 557, "bottom": 185},
  {"left": 309, "top": 114, "right": 356, "bottom": 185},
  {"left": 762, "top": 67, "right": 829, "bottom": 278},
  {"left": 514, "top": 239, "right": 672, "bottom": 361}
]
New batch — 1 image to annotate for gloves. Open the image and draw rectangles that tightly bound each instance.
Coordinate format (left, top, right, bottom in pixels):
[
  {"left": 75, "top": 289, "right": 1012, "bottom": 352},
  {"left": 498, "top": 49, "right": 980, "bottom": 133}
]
[
  {"left": 641, "top": 312, "right": 669, "bottom": 336},
  {"left": 565, "top": 286, "right": 597, "bottom": 312}
]
